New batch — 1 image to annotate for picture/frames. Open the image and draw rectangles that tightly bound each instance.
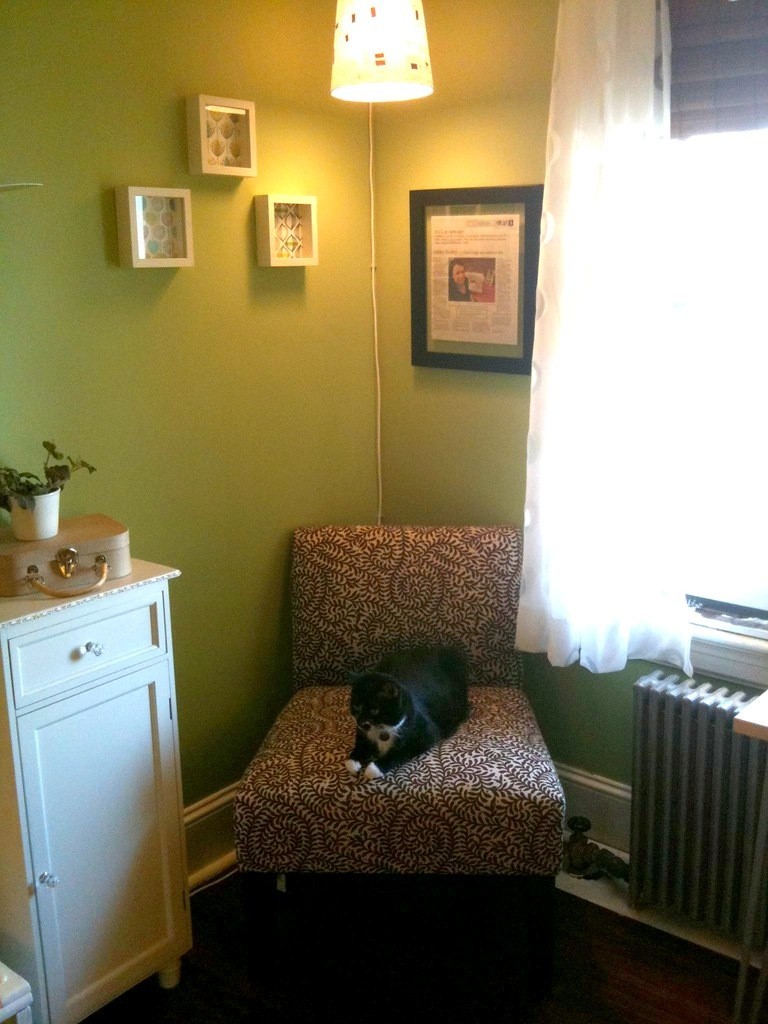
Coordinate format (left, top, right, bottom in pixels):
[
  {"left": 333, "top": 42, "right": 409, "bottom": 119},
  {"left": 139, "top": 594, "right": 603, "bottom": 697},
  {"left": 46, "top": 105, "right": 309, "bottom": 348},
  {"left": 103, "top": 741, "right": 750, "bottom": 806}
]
[{"left": 407, "top": 184, "right": 544, "bottom": 374}]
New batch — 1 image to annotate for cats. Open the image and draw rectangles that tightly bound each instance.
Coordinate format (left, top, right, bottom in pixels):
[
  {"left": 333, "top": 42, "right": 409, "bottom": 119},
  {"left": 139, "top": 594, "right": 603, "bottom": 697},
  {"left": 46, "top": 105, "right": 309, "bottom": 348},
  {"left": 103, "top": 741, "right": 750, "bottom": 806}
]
[{"left": 344, "top": 646, "right": 470, "bottom": 780}]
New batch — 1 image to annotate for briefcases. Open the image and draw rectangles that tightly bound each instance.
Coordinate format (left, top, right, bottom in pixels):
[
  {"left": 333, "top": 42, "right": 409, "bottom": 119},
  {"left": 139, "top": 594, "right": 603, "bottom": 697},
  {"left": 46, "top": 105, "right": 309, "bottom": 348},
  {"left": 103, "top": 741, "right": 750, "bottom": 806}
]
[{"left": 0, "top": 513, "right": 131, "bottom": 597}]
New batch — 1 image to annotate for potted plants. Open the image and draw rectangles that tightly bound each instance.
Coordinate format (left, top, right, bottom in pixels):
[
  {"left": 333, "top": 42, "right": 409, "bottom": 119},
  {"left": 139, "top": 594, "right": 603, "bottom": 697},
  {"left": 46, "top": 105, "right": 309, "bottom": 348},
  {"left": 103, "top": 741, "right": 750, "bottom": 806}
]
[{"left": 0, "top": 437, "right": 98, "bottom": 542}]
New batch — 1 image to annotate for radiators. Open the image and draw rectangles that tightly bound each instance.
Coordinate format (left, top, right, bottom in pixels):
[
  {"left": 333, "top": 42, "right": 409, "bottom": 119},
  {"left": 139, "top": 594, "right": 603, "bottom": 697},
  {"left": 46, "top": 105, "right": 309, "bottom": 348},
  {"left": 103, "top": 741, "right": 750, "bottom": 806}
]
[{"left": 628, "top": 667, "right": 768, "bottom": 956}]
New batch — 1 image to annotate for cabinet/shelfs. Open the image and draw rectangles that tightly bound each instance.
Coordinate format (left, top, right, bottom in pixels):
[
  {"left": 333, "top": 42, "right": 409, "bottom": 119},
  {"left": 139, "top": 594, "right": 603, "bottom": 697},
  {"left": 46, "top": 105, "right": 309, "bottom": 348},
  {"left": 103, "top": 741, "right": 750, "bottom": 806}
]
[{"left": 0, "top": 558, "right": 193, "bottom": 1024}]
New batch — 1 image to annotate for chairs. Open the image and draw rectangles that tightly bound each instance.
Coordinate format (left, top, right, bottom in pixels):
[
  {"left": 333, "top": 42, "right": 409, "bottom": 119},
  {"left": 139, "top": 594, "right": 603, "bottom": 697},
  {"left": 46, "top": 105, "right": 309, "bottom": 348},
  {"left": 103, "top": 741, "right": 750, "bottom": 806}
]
[{"left": 232, "top": 524, "right": 565, "bottom": 901}]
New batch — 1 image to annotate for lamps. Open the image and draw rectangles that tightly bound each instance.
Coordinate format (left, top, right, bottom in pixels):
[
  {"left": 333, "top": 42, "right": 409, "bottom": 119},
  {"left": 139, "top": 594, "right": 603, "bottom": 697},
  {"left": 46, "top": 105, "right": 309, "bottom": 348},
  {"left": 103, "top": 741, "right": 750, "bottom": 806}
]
[{"left": 330, "top": 0, "right": 435, "bottom": 104}]
